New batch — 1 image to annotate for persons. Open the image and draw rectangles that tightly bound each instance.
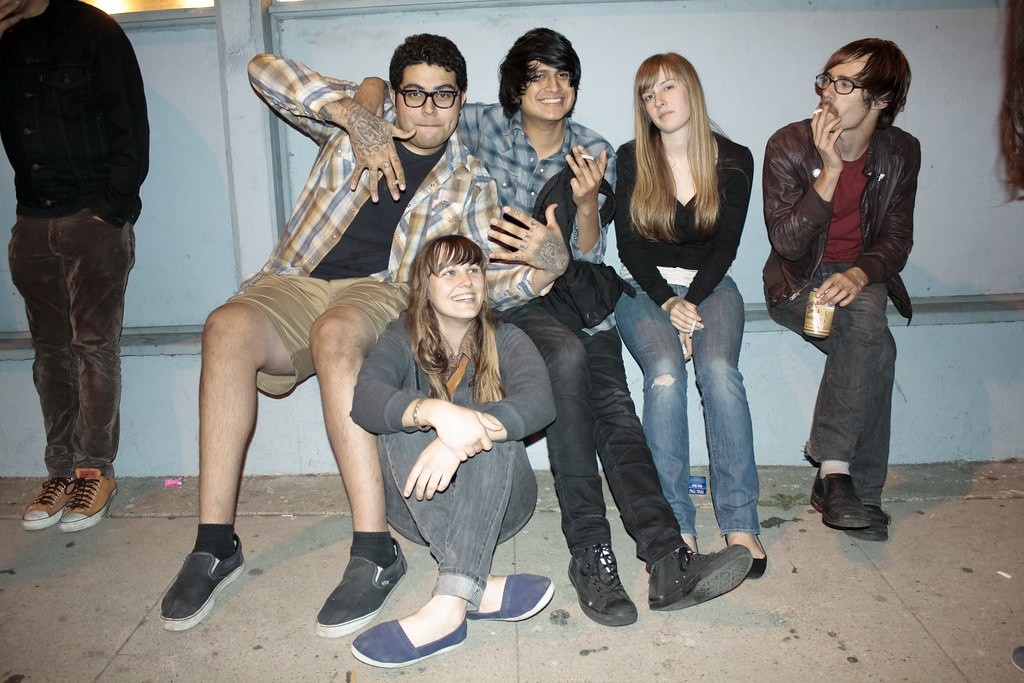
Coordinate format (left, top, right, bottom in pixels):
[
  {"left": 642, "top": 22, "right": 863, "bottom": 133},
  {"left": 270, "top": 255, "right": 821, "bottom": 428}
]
[
  {"left": 0, "top": 0, "right": 151, "bottom": 533},
  {"left": 350, "top": 28, "right": 754, "bottom": 627},
  {"left": 762, "top": 37, "right": 922, "bottom": 542},
  {"left": 159, "top": 33, "right": 571, "bottom": 638},
  {"left": 349, "top": 234, "right": 557, "bottom": 669},
  {"left": 612, "top": 51, "right": 768, "bottom": 579}
]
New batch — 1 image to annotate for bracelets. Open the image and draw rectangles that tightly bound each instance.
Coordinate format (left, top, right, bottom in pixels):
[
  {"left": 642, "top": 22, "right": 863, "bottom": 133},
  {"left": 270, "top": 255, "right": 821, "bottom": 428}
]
[{"left": 412, "top": 397, "right": 432, "bottom": 431}]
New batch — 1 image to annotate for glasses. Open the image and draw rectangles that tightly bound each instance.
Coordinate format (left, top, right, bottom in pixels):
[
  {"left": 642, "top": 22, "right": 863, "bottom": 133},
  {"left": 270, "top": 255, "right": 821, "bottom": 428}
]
[
  {"left": 394, "top": 85, "right": 461, "bottom": 109},
  {"left": 815, "top": 73, "right": 865, "bottom": 95}
]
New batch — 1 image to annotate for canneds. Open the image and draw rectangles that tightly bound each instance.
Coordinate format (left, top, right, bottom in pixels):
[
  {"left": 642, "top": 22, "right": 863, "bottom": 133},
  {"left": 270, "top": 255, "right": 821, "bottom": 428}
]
[{"left": 804, "top": 288, "right": 835, "bottom": 339}]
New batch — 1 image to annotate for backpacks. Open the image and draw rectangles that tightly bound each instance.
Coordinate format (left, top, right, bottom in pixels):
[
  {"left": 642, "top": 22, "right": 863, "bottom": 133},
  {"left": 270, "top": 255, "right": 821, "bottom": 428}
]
[{"left": 533, "top": 164, "right": 636, "bottom": 334}]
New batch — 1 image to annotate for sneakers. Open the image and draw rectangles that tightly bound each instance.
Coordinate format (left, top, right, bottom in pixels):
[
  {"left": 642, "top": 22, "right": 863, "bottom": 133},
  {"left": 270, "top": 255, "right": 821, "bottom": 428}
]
[
  {"left": 160, "top": 534, "right": 244, "bottom": 630},
  {"left": 351, "top": 617, "right": 468, "bottom": 669},
  {"left": 648, "top": 544, "right": 753, "bottom": 611},
  {"left": 21, "top": 468, "right": 118, "bottom": 533},
  {"left": 466, "top": 574, "right": 556, "bottom": 622},
  {"left": 313, "top": 538, "right": 409, "bottom": 638},
  {"left": 569, "top": 543, "right": 637, "bottom": 627}
]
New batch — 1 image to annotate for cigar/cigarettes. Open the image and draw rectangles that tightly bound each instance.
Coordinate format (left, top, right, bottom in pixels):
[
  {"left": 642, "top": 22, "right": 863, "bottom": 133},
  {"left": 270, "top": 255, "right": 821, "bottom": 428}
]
[
  {"left": 580, "top": 154, "right": 594, "bottom": 161},
  {"left": 392, "top": 179, "right": 399, "bottom": 185},
  {"left": 812, "top": 109, "right": 823, "bottom": 114},
  {"left": 688, "top": 319, "right": 697, "bottom": 338}
]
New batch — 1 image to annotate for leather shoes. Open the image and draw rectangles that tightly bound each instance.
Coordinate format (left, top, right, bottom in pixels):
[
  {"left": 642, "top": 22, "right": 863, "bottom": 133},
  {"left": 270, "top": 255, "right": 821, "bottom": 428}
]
[
  {"left": 810, "top": 468, "right": 888, "bottom": 542},
  {"left": 724, "top": 533, "right": 768, "bottom": 579}
]
